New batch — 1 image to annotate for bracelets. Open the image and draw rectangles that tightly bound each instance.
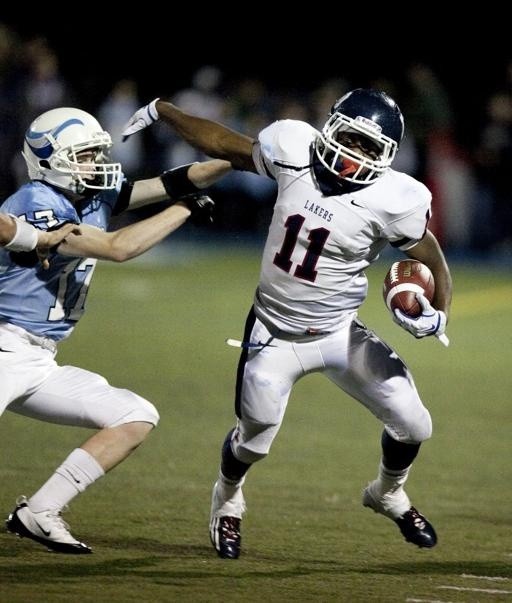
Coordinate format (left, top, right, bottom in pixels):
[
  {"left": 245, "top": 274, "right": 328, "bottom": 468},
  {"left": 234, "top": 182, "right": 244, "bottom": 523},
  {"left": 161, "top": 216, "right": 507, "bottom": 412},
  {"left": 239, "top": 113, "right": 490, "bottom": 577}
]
[
  {"left": 159, "top": 163, "right": 199, "bottom": 199},
  {"left": 6, "top": 214, "right": 38, "bottom": 253}
]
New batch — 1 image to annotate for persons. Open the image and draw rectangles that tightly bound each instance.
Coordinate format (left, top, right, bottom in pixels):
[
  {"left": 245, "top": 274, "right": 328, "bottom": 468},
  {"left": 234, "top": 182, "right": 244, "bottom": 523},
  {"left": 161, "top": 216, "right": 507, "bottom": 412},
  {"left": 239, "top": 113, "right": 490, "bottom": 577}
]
[
  {"left": 0, "top": 105, "right": 245, "bottom": 554},
  {"left": 1, "top": 210, "right": 81, "bottom": 270},
  {"left": 1, "top": 43, "right": 511, "bottom": 258},
  {"left": 120, "top": 89, "right": 453, "bottom": 559}
]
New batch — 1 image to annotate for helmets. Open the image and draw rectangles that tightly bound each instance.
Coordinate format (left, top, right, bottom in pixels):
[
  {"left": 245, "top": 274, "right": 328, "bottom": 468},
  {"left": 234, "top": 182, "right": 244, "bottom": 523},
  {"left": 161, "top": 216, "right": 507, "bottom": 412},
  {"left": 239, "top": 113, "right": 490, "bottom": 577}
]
[
  {"left": 310, "top": 87, "right": 406, "bottom": 195},
  {"left": 22, "top": 107, "right": 127, "bottom": 196}
]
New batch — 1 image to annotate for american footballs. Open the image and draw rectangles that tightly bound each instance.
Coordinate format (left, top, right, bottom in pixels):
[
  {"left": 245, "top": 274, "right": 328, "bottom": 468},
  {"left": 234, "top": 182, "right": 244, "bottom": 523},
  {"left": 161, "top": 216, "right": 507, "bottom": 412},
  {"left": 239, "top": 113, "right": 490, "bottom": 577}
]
[{"left": 383, "top": 259, "right": 435, "bottom": 317}]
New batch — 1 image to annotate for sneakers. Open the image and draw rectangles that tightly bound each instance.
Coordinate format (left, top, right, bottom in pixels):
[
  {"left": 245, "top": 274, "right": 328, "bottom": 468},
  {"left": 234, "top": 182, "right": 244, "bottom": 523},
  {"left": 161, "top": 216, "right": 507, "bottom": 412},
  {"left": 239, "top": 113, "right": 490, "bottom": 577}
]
[
  {"left": 4, "top": 495, "right": 93, "bottom": 554},
  {"left": 362, "top": 479, "right": 437, "bottom": 548},
  {"left": 209, "top": 479, "right": 247, "bottom": 559}
]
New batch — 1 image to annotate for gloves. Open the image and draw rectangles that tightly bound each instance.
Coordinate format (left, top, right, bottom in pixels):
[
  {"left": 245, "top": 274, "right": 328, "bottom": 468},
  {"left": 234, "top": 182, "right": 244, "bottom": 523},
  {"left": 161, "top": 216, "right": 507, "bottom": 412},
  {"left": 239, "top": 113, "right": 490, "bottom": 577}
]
[
  {"left": 183, "top": 191, "right": 221, "bottom": 233},
  {"left": 121, "top": 97, "right": 161, "bottom": 142},
  {"left": 392, "top": 292, "right": 450, "bottom": 347}
]
